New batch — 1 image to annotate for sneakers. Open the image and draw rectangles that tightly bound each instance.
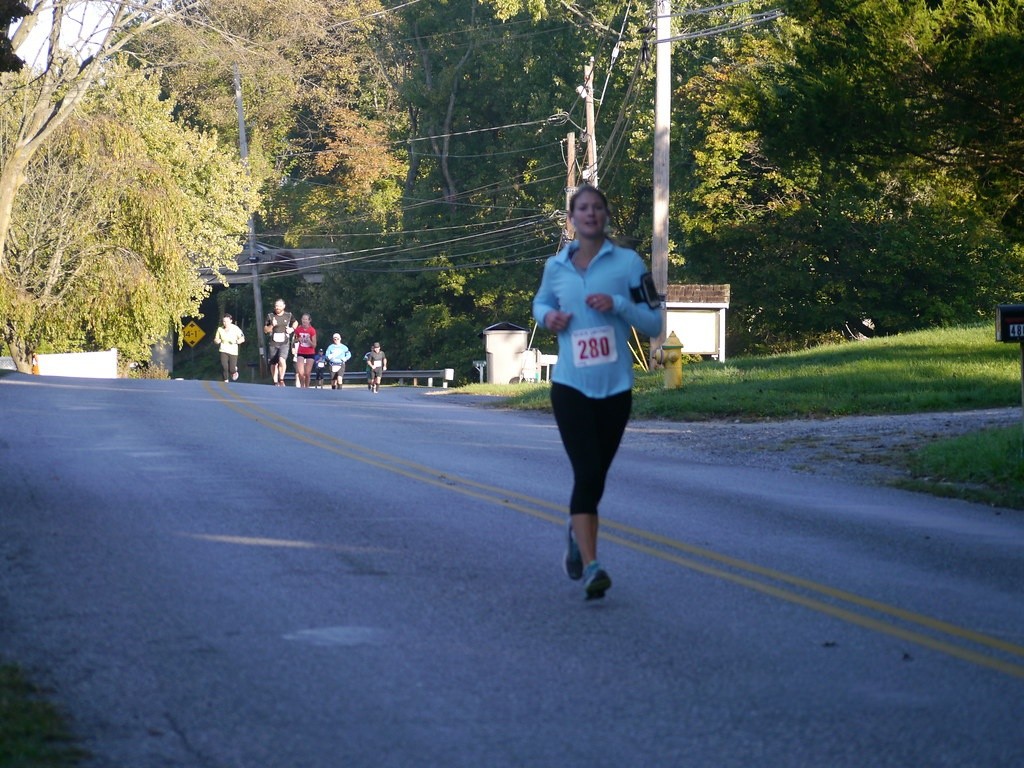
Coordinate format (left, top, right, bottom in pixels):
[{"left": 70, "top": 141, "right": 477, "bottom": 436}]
[
  {"left": 563, "top": 518, "right": 585, "bottom": 580},
  {"left": 583, "top": 565, "right": 611, "bottom": 600}
]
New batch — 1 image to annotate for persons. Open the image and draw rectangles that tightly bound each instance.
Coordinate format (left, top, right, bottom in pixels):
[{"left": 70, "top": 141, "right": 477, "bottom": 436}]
[
  {"left": 214, "top": 313, "right": 245, "bottom": 382},
  {"left": 532, "top": 183, "right": 663, "bottom": 603},
  {"left": 263, "top": 298, "right": 328, "bottom": 394},
  {"left": 324, "top": 332, "right": 352, "bottom": 390},
  {"left": 363, "top": 341, "right": 388, "bottom": 394}
]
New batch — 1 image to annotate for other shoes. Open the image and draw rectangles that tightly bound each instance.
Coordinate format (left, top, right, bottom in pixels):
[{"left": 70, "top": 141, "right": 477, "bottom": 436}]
[
  {"left": 368, "top": 384, "right": 371, "bottom": 390},
  {"left": 274, "top": 383, "right": 280, "bottom": 386},
  {"left": 225, "top": 379, "right": 228, "bottom": 383},
  {"left": 280, "top": 381, "right": 285, "bottom": 386},
  {"left": 232, "top": 366, "right": 239, "bottom": 380},
  {"left": 372, "top": 384, "right": 376, "bottom": 392}
]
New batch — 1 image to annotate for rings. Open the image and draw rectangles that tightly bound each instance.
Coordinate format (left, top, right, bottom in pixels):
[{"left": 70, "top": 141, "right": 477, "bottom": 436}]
[{"left": 593, "top": 297, "right": 598, "bottom": 302}]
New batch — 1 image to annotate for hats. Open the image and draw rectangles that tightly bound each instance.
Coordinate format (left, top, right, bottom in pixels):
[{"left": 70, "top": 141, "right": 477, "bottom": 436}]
[
  {"left": 319, "top": 349, "right": 323, "bottom": 352},
  {"left": 333, "top": 333, "right": 341, "bottom": 339},
  {"left": 374, "top": 342, "right": 380, "bottom": 348}
]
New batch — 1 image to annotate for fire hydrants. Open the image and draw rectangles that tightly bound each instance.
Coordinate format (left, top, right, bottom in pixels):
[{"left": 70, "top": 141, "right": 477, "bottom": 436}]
[{"left": 652, "top": 331, "right": 683, "bottom": 392}]
